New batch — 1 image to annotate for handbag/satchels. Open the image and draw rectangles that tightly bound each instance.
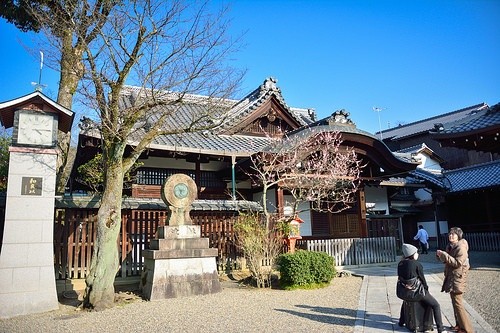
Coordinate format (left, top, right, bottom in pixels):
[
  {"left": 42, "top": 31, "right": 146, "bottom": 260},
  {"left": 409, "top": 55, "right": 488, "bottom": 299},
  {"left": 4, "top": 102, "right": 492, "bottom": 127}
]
[{"left": 425, "top": 241, "right": 430, "bottom": 249}]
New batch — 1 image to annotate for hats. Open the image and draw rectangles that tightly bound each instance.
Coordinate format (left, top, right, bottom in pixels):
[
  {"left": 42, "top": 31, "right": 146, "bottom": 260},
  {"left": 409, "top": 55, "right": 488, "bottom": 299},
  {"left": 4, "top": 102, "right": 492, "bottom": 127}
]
[{"left": 403, "top": 244, "right": 418, "bottom": 258}]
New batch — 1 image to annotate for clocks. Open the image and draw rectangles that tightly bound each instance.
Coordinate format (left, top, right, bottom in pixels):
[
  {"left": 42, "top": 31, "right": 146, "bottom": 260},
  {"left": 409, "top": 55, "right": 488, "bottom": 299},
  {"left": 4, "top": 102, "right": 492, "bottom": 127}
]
[{"left": 17, "top": 112, "right": 53, "bottom": 145}]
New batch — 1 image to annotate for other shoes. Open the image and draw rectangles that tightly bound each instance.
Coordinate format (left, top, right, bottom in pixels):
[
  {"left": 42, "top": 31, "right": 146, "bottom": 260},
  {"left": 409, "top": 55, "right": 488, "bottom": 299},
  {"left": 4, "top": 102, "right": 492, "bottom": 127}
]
[
  {"left": 438, "top": 327, "right": 446, "bottom": 332},
  {"left": 399, "top": 321, "right": 406, "bottom": 327},
  {"left": 450, "top": 325, "right": 467, "bottom": 333},
  {"left": 420, "top": 251, "right": 428, "bottom": 254}
]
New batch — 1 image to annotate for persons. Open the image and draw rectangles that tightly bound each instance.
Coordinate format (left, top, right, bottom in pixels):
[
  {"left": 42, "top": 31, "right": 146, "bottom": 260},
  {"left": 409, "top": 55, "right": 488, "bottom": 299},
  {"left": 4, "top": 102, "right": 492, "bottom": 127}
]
[
  {"left": 395, "top": 242, "right": 447, "bottom": 333},
  {"left": 433, "top": 227, "right": 474, "bottom": 333},
  {"left": 414, "top": 224, "right": 430, "bottom": 255}
]
